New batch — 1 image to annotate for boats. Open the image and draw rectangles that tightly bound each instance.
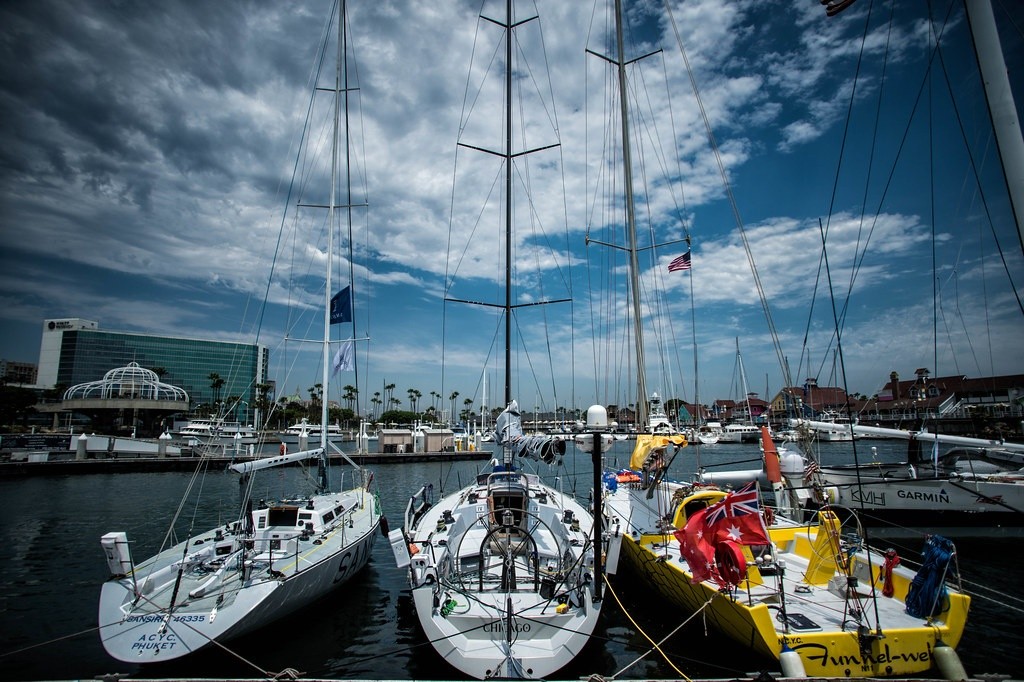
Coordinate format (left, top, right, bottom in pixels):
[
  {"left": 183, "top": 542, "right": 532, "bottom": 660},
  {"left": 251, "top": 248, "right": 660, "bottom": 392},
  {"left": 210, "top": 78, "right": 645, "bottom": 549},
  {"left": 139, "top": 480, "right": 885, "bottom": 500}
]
[
  {"left": 694, "top": 0, "right": 1024, "bottom": 544},
  {"left": 273, "top": 417, "right": 343, "bottom": 443},
  {"left": 165, "top": 412, "right": 260, "bottom": 445}
]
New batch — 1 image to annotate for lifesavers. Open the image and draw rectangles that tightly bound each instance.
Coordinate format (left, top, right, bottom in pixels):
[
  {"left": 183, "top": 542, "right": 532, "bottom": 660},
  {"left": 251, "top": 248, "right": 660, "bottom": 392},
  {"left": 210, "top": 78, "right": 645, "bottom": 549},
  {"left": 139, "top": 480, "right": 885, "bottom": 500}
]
[{"left": 714, "top": 538, "right": 748, "bottom": 584}]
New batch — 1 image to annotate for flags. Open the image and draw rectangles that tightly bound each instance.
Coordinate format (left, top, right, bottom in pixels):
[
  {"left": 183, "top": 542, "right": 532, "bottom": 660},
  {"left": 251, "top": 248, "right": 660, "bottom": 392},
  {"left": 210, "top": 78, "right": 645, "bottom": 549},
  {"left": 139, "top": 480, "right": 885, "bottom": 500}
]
[
  {"left": 668, "top": 251, "right": 691, "bottom": 272},
  {"left": 329, "top": 285, "right": 352, "bottom": 324},
  {"left": 332, "top": 337, "right": 353, "bottom": 377},
  {"left": 673, "top": 480, "right": 769, "bottom": 584},
  {"left": 806, "top": 456, "right": 818, "bottom": 484}
]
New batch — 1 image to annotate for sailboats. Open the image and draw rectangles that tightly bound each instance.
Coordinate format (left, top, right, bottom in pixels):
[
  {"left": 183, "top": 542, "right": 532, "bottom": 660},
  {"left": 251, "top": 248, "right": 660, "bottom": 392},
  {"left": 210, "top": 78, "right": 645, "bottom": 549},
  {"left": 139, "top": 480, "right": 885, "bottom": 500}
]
[
  {"left": 589, "top": 0, "right": 970, "bottom": 682},
  {"left": 97, "top": 0, "right": 379, "bottom": 663},
  {"left": 387, "top": 0, "right": 623, "bottom": 681}
]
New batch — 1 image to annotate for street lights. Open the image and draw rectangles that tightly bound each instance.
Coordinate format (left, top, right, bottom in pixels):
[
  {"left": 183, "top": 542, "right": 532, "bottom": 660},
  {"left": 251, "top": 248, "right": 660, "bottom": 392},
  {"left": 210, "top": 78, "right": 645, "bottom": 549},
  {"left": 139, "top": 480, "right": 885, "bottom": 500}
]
[
  {"left": 195, "top": 405, "right": 200, "bottom": 414},
  {"left": 245, "top": 402, "right": 248, "bottom": 426},
  {"left": 362, "top": 409, "right": 373, "bottom": 422}
]
